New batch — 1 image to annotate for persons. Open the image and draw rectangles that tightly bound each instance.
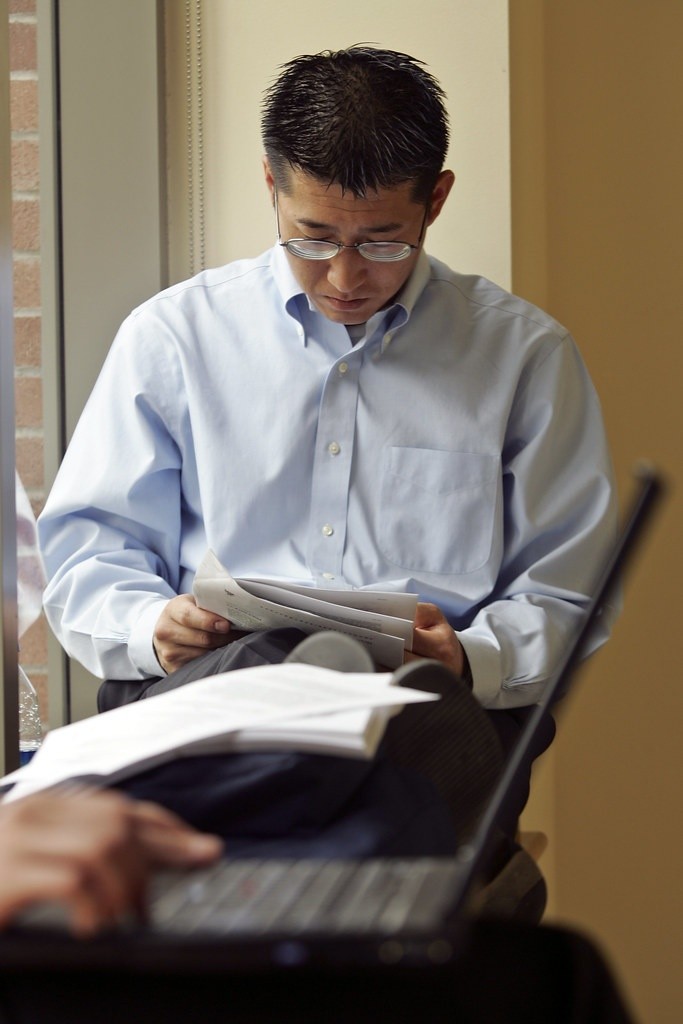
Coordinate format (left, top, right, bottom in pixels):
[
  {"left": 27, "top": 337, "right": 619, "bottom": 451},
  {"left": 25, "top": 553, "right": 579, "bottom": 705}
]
[
  {"left": 34, "top": 50, "right": 617, "bottom": 869},
  {"left": 0, "top": 785, "right": 638, "bottom": 1024}
]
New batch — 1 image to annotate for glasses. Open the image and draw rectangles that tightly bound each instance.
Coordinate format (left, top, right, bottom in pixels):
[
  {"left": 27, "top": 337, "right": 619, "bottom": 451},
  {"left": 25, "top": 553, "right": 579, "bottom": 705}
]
[{"left": 272, "top": 177, "right": 432, "bottom": 263}]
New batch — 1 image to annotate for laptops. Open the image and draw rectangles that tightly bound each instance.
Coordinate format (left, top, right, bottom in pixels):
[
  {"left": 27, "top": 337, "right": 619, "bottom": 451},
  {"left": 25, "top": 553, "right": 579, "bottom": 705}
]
[{"left": 0, "top": 462, "right": 660, "bottom": 966}]
